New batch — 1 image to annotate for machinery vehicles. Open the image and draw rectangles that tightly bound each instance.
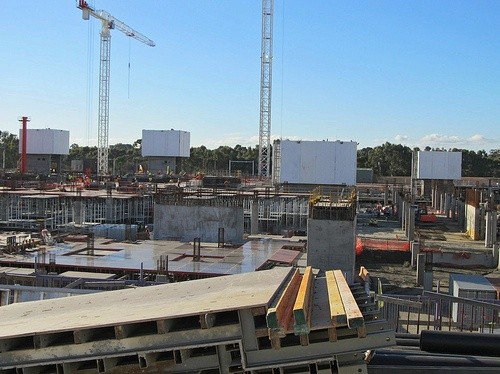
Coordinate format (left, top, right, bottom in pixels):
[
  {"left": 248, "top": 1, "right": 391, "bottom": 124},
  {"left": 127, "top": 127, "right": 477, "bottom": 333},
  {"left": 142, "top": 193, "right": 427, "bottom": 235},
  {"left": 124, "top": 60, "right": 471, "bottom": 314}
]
[{"left": 416, "top": 205, "right": 436, "bottom": 228}]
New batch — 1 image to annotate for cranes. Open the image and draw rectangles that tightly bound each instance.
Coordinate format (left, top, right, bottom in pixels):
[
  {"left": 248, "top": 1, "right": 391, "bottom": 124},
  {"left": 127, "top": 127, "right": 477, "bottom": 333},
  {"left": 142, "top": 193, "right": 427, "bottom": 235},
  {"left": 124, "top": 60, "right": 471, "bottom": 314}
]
[{"left": 75, "top": 0, "right": 155, "bottom": 175}]
